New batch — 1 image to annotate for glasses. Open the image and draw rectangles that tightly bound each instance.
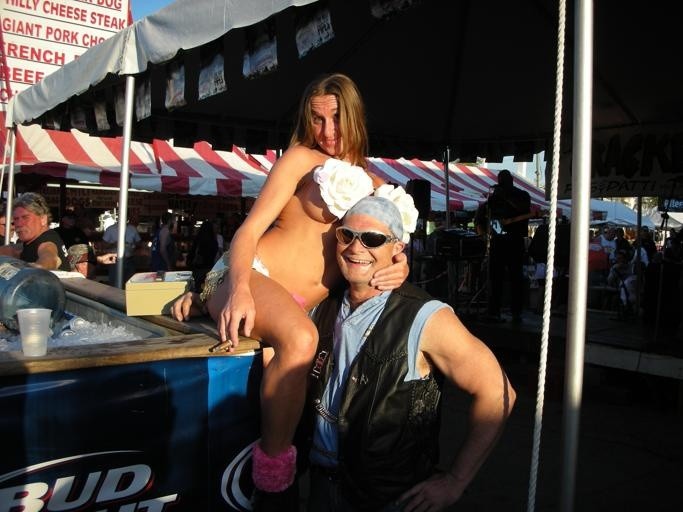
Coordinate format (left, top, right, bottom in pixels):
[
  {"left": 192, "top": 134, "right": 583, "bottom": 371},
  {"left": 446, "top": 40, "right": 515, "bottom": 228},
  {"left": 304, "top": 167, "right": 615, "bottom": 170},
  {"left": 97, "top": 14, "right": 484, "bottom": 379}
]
[
  {"left": 333, "top": 225, "right": 397, "bottom": 250},
  {"left": 1, "top": 221, "right": 15, "bottom": 228}
]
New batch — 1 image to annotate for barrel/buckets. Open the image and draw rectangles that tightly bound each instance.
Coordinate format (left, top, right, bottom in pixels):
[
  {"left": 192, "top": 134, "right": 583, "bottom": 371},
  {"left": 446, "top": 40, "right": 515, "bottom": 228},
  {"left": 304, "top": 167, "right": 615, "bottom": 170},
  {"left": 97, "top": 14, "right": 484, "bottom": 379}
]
[
  {"left": 412, "top": 253, "right": 447, "bottom": 301},
  {"left": 1, "top": 256, "right": 66, "bottom": 335}
]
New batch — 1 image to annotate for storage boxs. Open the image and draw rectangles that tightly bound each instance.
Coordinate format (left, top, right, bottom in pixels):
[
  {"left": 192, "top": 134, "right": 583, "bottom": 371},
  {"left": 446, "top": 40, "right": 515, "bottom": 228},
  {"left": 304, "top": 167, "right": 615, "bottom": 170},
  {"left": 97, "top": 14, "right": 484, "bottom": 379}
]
[{"left": 124, "top": 270, "right": 196, "bottom": 317}]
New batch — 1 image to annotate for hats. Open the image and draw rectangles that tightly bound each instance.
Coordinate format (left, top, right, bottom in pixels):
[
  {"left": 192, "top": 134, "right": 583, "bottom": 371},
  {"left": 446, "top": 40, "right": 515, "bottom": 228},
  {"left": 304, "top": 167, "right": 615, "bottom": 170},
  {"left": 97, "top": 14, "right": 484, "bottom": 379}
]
[{"left": 345, "top": 196, "right": 404, "bottom": 240}]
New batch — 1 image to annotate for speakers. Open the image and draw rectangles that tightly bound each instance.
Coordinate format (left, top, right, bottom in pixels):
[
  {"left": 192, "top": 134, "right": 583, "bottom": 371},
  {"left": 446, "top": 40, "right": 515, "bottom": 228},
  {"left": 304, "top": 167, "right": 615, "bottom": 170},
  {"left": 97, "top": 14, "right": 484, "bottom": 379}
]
[{"left": 406, "top": 178, "right": 431, "bottom": 219}]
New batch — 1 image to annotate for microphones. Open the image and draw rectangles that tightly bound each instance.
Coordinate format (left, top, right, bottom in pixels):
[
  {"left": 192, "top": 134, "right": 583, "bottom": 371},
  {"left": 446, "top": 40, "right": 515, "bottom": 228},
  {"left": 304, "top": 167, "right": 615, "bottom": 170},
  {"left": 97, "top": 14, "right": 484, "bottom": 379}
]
[{"left": 489, "top": 184, "right": 501, "bottom": 188}]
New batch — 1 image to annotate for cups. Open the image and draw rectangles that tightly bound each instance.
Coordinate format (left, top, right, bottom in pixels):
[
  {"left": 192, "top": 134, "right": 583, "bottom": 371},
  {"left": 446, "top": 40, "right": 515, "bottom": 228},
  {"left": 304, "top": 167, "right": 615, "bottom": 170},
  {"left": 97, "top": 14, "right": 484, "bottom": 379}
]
[{"left": 16, "top": 308, "right": 51, "bottom": 357}]
